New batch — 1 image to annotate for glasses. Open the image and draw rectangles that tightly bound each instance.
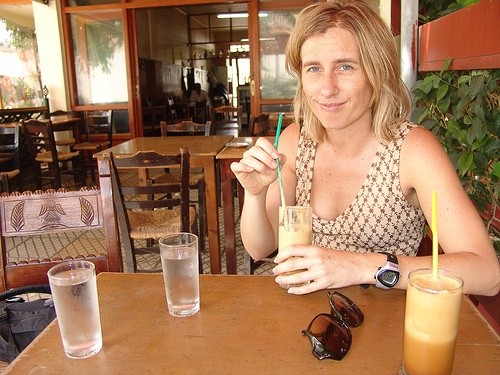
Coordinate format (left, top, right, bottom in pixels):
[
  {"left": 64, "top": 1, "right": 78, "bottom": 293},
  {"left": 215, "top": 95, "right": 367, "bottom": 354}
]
[{"left": 301, "top": 291, "right": 364, "bottom": 361}]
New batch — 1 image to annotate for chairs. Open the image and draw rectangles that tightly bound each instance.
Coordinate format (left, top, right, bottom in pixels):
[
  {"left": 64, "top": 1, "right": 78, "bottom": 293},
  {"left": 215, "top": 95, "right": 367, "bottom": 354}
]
[
  {"left": 0, "top": 108, "right": 113, "bottom": 195},
  {"left": 150, "top": 121, "right": 211, "bottom": 235},
  {"left": 0, "top": 187, "right": 109, "bottom": 290},
  {"left": 142, "top": 101, "right": 197, "bottom": 128},
  {"left": 209, "top": 105, "right": 243, "bottom": 137},
  {"left": 108, "top": 150, "right": 206, "bottom": 271},
  {"left": 231, "top": 112, "right": 270, "bottom": 198}
]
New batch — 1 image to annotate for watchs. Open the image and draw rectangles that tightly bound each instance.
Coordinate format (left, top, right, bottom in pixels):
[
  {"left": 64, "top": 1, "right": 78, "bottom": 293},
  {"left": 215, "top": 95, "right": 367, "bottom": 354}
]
[{"left": 374, "top": 252, "right": 401, "bottom": 291}]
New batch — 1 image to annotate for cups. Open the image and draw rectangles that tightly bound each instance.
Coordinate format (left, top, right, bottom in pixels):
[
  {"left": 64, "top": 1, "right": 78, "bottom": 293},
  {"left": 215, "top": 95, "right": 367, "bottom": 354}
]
[
  {"left": 279, "top": 205, "right": 312, "bottom": 289},
  {"left": 47, "top": 261, "right": 102, "bottom": 359},
  {"left": 159, "top": 232, "right": 200, "bottom": 317},
  {"left": 398, "top": 268, "right": 464, "bottom": 375}
]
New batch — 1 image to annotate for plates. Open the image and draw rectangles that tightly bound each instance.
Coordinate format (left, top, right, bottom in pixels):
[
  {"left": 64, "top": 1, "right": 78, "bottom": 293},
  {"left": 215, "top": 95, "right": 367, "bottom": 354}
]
[{"left": 226, "top": 142, "right": 248, "bottom": 147}]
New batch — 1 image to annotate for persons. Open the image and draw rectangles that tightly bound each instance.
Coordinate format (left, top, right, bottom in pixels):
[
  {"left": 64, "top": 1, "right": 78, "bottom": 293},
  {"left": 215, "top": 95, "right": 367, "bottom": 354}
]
[
  {"left": 207, "top": 71, "right": 217, "bottom": 98},
  {"left": 190, "top": 83, "right": 208, "bottom": 102},
  {"left": 230, "top": 0, "right": 500, "bottom": 296},
  {"left": 212, "top": 83, "right": 229, "bottom": 106}
]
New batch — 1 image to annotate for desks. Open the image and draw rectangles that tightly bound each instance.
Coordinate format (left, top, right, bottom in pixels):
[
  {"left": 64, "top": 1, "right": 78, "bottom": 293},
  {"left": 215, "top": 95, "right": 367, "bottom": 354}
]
[
  {"left": 0, "top": 118, "right": 86, "bottom": 186},
  {"left": 0, "top": 218, "right": 250, "bottom": 272},
  {"left": 264, "top": 110, "right": 304, "bottom": 134},
  {"left": 94, "top": 134, "right": 233, "bottom": 275},
  {"left": 216, "top": 136, "right": 277, "bottom": 274},
  {"left": 0, "top": 271, "right": 500, "bottom": 375}
]
